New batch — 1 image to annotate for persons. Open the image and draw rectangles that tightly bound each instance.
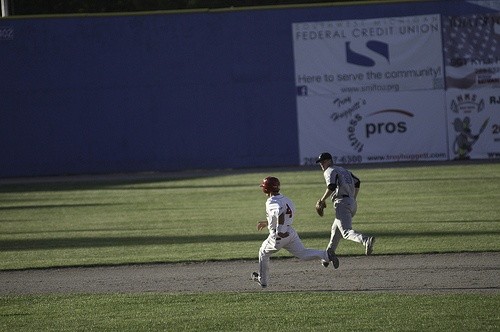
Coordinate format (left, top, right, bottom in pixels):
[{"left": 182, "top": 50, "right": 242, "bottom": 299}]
[
  {"left": 250, "top": 176, "right": 340, "bottom": 289},
  {"left": 315, "top": 152, "right": 376, "bottom": 268}
]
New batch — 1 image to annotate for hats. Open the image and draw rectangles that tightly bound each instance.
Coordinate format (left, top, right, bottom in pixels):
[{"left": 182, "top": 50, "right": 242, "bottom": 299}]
[{"left": 316, "top": 152, "right": 332, "bottom": 163}]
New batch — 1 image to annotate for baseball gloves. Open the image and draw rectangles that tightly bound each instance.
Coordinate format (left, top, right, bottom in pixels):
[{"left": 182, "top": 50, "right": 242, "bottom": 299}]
[{"left": 315, "top": 199, "right": 327, "bottom": 217}]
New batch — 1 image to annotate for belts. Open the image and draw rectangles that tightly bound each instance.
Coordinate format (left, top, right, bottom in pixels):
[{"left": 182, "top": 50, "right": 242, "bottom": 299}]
[{"left": 334, "top": 194, "right": 350, "bottom": 201}]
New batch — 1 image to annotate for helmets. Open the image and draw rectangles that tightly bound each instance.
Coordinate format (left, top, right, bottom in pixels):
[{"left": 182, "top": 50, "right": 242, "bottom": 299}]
[{"left": 260, "top": 177, "right": 281, "bottom": 194}]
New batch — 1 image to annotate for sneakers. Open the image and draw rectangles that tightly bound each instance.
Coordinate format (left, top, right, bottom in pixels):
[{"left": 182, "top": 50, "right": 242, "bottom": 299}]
[
  {"left": 327, "top": 248, "right": 339, "bottom": 269},
  {"left": 251, "top": 272, "right": 267, "bottom": 288},
  {"left": 322, "top": 259, "right": 329, "bottom": 268},
  {"left": 365, "top": 236, "right": 375, "bottom": 255}
]
